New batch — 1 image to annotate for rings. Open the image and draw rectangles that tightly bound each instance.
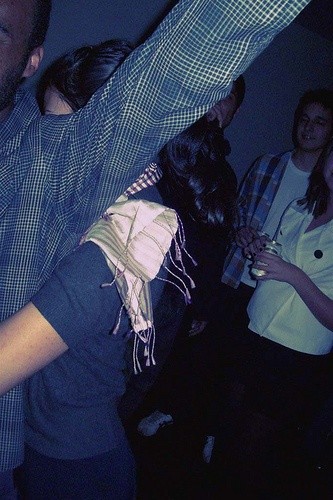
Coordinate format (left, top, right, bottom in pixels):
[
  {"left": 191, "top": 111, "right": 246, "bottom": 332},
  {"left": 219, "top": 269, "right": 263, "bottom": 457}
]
[{"left": 195, "top": 321, "right": 202, "bottom": 327}]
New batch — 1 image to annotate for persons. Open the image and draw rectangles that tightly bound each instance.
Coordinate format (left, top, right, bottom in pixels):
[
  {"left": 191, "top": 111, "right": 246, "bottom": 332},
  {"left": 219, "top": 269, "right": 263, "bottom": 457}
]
[
  {"left": 0, "top": 0, "right": 311, "bottom": 500},
  {"left": 0, "top": 37, "right": 333, "bottom": 500}
]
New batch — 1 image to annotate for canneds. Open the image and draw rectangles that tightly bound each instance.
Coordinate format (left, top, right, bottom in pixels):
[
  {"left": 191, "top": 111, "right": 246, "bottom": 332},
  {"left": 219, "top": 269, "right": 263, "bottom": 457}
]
[{"left": 251, "top": 239, "right": 282, "bottom": 277}]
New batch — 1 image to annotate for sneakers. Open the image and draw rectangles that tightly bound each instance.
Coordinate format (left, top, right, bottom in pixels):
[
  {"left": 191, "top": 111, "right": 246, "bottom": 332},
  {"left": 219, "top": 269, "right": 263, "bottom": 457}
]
[
  {"left": 137, "top": 410, "right": 175, "bottom": 438},
  {"left": 200, "top": 434, "right": 215, "bottom": 463}
]
